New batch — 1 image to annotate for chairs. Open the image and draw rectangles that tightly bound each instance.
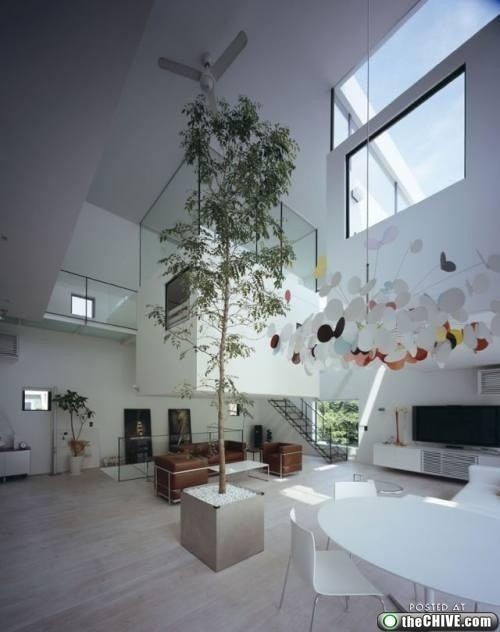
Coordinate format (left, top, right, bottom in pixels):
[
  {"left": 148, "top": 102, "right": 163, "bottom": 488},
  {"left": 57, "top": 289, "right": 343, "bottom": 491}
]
[
  {"left": 261, "top": 441, "right": 303, "bottom": 480},
  {"left": 278, "top": 507, "right": 387, "bottom": 632},
  {"left": 153, "top": 451, "right": 209, "bottom": 504},
  {"left": 332, "top": 480, "right": 379, "bottom": 499}
]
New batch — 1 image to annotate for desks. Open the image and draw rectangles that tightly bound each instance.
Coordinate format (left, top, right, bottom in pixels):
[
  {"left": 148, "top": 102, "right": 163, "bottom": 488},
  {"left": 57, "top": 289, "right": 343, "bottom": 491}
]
[
  {"left": 317, "top": 495, "right": 500, "bottom": 631},
  {"left": 0, "top": 446, "right": 33, "bottom": 483}
]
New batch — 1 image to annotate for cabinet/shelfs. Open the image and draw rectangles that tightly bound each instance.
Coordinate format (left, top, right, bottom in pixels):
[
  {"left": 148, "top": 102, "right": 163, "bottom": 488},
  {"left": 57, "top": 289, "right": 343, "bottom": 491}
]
[{"left": 373, "top": 443, "right": 500, "bottom": 482}]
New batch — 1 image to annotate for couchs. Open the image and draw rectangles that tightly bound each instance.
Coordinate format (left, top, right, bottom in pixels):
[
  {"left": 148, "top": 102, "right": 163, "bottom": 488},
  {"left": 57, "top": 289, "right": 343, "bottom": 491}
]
[{"left": 177, "top": 439, "right": 248, "bottom": 477}]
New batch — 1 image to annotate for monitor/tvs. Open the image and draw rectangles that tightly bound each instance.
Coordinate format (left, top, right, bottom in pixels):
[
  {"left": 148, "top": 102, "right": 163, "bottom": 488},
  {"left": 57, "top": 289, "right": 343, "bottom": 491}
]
[{"left": 411, "top": 405, "right": 500, "bottom": 449}]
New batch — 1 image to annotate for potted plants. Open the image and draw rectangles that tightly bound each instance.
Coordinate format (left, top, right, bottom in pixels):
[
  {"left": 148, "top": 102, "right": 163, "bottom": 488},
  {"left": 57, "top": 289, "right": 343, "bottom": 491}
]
[
  {"left": 51, "top": 390, "right": 96, "bottom": 476},
  {"left": 147, "top": 93, "right": 301, "bottom": 574}
]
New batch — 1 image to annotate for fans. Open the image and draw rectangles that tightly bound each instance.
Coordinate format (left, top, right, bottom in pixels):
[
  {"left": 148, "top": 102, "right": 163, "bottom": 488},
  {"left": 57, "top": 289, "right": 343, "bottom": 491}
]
[{"left": 156, "top": 30, "right": 249, "bottom": 118}]
